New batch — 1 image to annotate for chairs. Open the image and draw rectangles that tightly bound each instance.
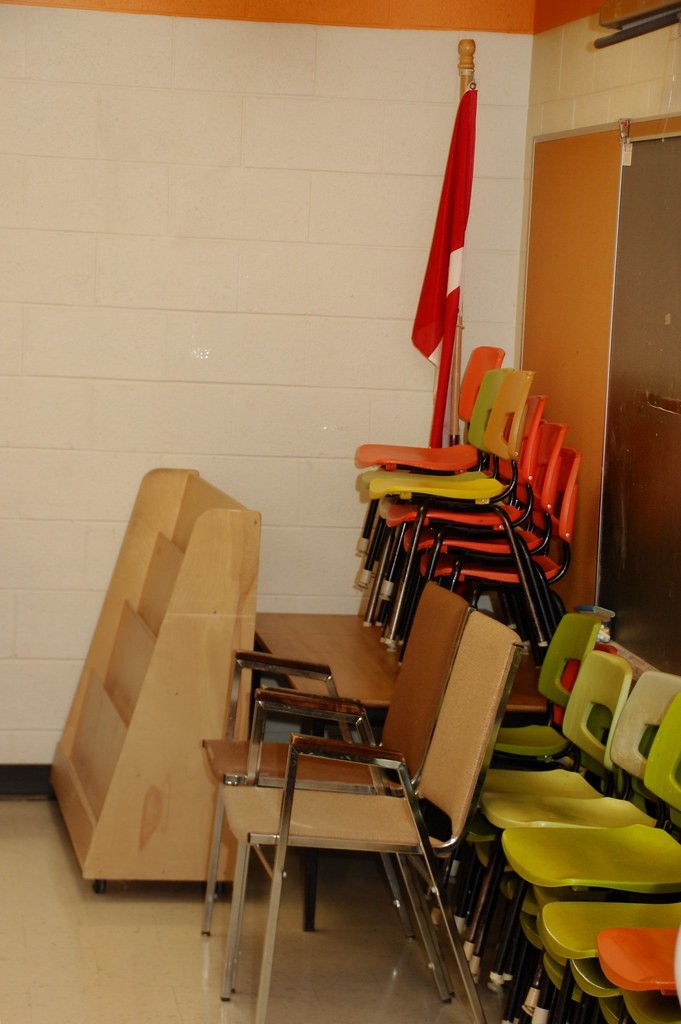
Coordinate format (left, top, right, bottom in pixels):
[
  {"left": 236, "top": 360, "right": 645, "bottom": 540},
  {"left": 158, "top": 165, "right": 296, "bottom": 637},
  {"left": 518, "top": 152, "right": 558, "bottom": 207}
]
[{"left": 202, "top": 345, "right": 681, "bottom": 1024}]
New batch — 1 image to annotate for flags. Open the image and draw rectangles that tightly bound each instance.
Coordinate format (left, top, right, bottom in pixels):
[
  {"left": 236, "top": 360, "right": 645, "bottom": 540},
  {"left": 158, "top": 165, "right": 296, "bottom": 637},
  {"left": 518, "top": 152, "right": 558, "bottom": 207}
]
[{"left": 412, "top": 79, "right": 477, "bottom": 449}]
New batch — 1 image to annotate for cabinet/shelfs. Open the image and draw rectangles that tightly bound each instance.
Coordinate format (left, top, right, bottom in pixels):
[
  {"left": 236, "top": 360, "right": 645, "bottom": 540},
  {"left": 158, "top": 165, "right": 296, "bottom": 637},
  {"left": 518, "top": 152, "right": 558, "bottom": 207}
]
[{"left": 52, "top": 470, "right": 262, "bottom": 897}]
